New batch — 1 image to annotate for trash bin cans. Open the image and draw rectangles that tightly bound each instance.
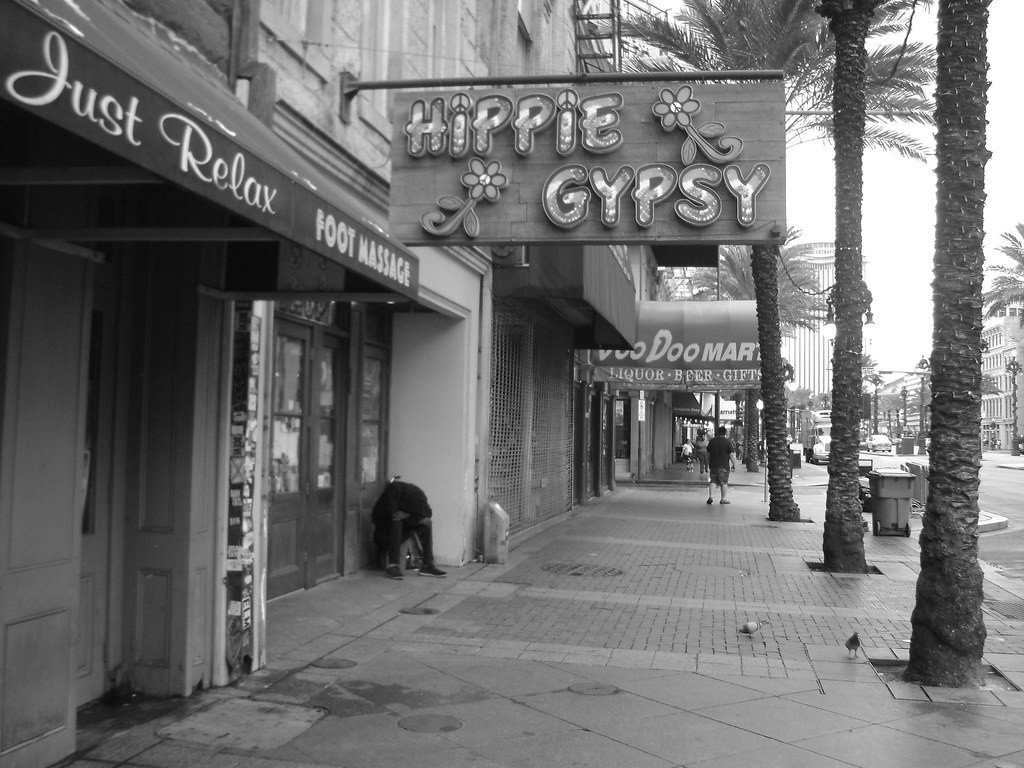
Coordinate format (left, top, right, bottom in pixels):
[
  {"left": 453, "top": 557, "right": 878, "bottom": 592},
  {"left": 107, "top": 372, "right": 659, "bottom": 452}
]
[
  {"left": 895, "top": 437, "right": 915, "bottom": 454},
  {"left": 482, "top": 500, "right": 510, "bottom": 564},
  {"left": 789, "top": 448, "right": 801, "bottom": 479},
  {"left": 866, "top": 466, "right": 917, "bottom": 538}
]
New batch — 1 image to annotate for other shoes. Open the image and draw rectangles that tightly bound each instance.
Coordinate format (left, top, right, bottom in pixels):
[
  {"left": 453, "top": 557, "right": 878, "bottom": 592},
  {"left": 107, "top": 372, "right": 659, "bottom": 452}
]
[
  {"left": 707, "top": 497, "right": 713, "bottom": 504},
  {"left": 720, "top": 500, "right": 730, "bottom": 504}
]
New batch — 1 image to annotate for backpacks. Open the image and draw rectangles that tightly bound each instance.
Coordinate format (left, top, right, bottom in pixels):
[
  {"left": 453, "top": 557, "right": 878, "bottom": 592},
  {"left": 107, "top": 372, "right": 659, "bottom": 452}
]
[{"left": 406, "top": 534, "right": 423, "bottom": 569}]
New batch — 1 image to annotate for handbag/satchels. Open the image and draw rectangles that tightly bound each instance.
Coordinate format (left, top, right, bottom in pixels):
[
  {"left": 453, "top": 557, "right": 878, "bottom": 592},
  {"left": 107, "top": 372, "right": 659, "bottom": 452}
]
[{"left": 689, "top": 453, "right": 692, "bottom": 456}]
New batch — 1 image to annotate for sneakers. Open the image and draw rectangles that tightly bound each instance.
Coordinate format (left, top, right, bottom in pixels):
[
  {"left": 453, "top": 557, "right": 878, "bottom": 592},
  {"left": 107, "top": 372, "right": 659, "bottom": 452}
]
[
  {"left": 419, "top": 565, "right": 447, "bottom": 577},
  {"left": 385, "top": 566, "right": 405, "bottom": 579}
]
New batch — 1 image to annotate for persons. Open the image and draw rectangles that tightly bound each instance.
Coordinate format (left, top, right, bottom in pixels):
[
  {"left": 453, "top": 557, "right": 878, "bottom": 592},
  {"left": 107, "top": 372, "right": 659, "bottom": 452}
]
[
  {"left": 370, "top": 481, "right": 448, "bottom": 580},
  {"left": 706, "top": 427, "right": 738, "bottom": 505},
  {"left": 680, "top": 439, "right": 695, "bottom": 472},
  {"left": 695, "top": 435, "right": 710, "bottom": 473}
]
